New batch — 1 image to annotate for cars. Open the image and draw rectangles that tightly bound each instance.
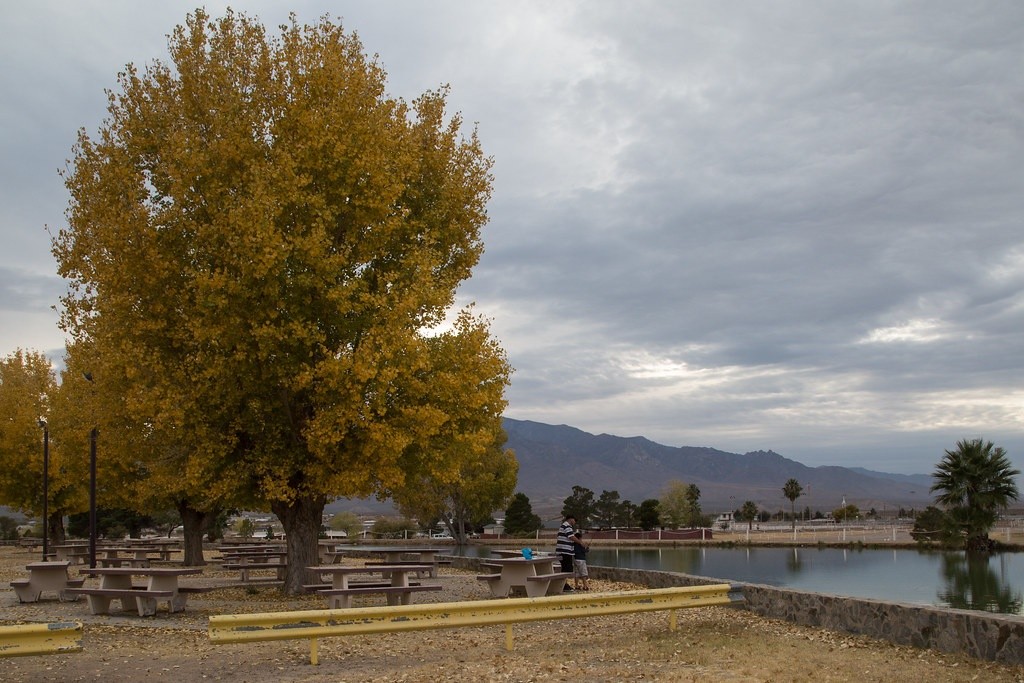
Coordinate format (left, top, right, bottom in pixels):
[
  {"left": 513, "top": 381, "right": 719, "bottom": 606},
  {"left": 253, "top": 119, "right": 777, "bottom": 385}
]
[
  {"left": 468, "top": 531, "right": 481, "bottom": 539},
  {"left": 432, "top": 534, "right": 453, "bottom": 541}
]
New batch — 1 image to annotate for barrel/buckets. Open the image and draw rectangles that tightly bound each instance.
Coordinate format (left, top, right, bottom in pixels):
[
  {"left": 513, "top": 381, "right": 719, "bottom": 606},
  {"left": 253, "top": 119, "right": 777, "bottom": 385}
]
[{"left": 522, "top": 547, "right": 532, "bottom": 560}]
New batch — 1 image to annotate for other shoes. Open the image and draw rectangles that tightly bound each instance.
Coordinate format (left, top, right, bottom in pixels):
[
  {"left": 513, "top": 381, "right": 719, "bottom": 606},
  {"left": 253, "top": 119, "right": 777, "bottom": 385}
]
[
  {"left": 575, "top": 587, "right": 580, "bottom": 591},
  {"left": 563, "top": 584, "right": 574, "bottom": 592},
  {"left": 583, "top": 586, "right": 590, "bottom": 592}
]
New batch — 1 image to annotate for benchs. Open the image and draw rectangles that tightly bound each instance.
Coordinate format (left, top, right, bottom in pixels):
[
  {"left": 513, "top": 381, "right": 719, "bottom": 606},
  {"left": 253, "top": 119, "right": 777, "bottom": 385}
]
[
  {"left": 528, "top": 572, "right": 577, "bottom": 583},
  {"left": 133, "top": 582, "right": 210, "bottom": 594},
  {"left": 366, "top": 561, "right": 453, "bottom": 566},
  {"left": 67, "top": 578, "right": 85, "bottom": 587},
  {"left": 211, "top": 552, "right": 283, "bottom": 568},
  {"left": 65, "top": 553, "right": 89, "bottom": 557},
  {"left": 325, "top": 551, "right": 346, "bottom": 555},
  {"left": 302, "top": 582, "right": 443, "bottom": 596},
  {"left": 477, "top": 573, "right": 501, "bottom": 581},
  {"left": 43, "top": 553, "right": 55, "bottom": 557},
  {"left": 65, "top": 588, "right": 173, "bottom": 597},
  {"left": 10, "top": 578, "right": 30, "bottom": 588},
  {"left": 95, "top": 547, "right": 181, "bottom": 563},
  {"left": 481, "top": 562, "right": 503, "bottom": 569}
]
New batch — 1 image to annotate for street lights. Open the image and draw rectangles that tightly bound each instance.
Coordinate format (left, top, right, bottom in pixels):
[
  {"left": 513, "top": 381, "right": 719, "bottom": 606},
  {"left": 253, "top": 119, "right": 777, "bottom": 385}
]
[
  {"left": 35, "top": 417, "right": 49, "bottom": 561},
  {"left": 78, "top": 368, "right": 97, "bottom": 576}
]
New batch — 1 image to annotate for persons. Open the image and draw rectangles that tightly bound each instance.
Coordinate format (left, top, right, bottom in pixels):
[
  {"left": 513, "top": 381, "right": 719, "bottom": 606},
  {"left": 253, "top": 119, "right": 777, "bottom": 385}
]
[{"left": 555, "top": 514, "right": 590, "bottom": 591}]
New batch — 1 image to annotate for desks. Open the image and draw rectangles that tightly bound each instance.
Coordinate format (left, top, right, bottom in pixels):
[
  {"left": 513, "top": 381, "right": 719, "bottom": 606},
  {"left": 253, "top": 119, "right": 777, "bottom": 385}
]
[
  {"left": 9, "top": 561, "right": 85, "bottom": 603},
  {"left": 318, "top": 542, "right": 347, "bottom": 565},
  {"left": 477, "top": 549, "right": 577, "bottom": 599},
  {"left": 47, "top": 545, "right": 89, "bottom": 566},
  {"left": 101, "top": 547, "right": 160, "bottom": 568},
  {"left": 64, "top": 568, "right": 204, "bottom": 617},
  {"left": 303, "top": 565, "right": 443, "bottom": 610},
  {"left": 126, "top": 538, "right": 159, "bottom": 550},
  {"left": 365, "top": 548, "right": 453, "bottom": 578},
  {"left": 65, "top": 540, "right": 91, "bottom": 545},
  {"left": 217, "top": 546, "right": 270, "bottom": 570},
  {"left": 132, "top": 541, "right": 180, "bottom": 561}
]
